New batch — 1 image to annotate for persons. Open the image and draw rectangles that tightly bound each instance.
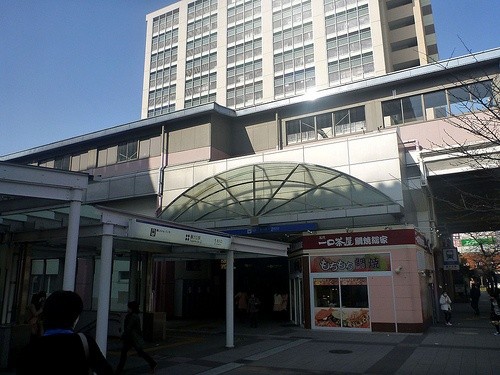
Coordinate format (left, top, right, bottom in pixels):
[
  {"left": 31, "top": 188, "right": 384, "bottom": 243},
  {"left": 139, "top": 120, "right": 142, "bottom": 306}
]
[
  {"left": 440, "top": 291, "right": 453, "bottom": 326},
  {"left": 15, "top": 291, "right": 117, "bottom": 375},
  {"left": 488, "top": 295, "right": 500, "bottom": 336},
  {"left": 235, "top": 289, "right": 261, "bottom": 329},
  {"left": 116, "top": 303, "right": 157, "bottom": 375},
  {"left": 470, "top": 285, "right": 481, "bottom": 315}
]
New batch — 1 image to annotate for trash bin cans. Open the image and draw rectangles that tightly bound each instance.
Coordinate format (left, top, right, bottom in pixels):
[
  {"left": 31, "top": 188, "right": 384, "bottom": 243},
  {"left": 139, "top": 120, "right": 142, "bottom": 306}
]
[{"left": 143, "top": 312, "right": 167, "bottom": 341}]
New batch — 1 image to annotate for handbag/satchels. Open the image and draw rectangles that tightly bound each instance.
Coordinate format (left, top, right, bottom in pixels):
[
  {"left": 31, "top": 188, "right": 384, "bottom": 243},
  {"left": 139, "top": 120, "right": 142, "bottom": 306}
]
[{"left": 134, "top": 336, "right": 144, "bottom": 350}]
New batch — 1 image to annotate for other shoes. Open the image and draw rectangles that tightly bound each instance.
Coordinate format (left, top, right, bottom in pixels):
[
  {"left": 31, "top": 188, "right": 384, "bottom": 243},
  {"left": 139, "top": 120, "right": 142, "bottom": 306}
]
[
  {"left": 150, "top": 362, "right": 156, "bottom": 371},
  {"left": 445, "top": 321, "right": 452, "bottom": 325}
]
